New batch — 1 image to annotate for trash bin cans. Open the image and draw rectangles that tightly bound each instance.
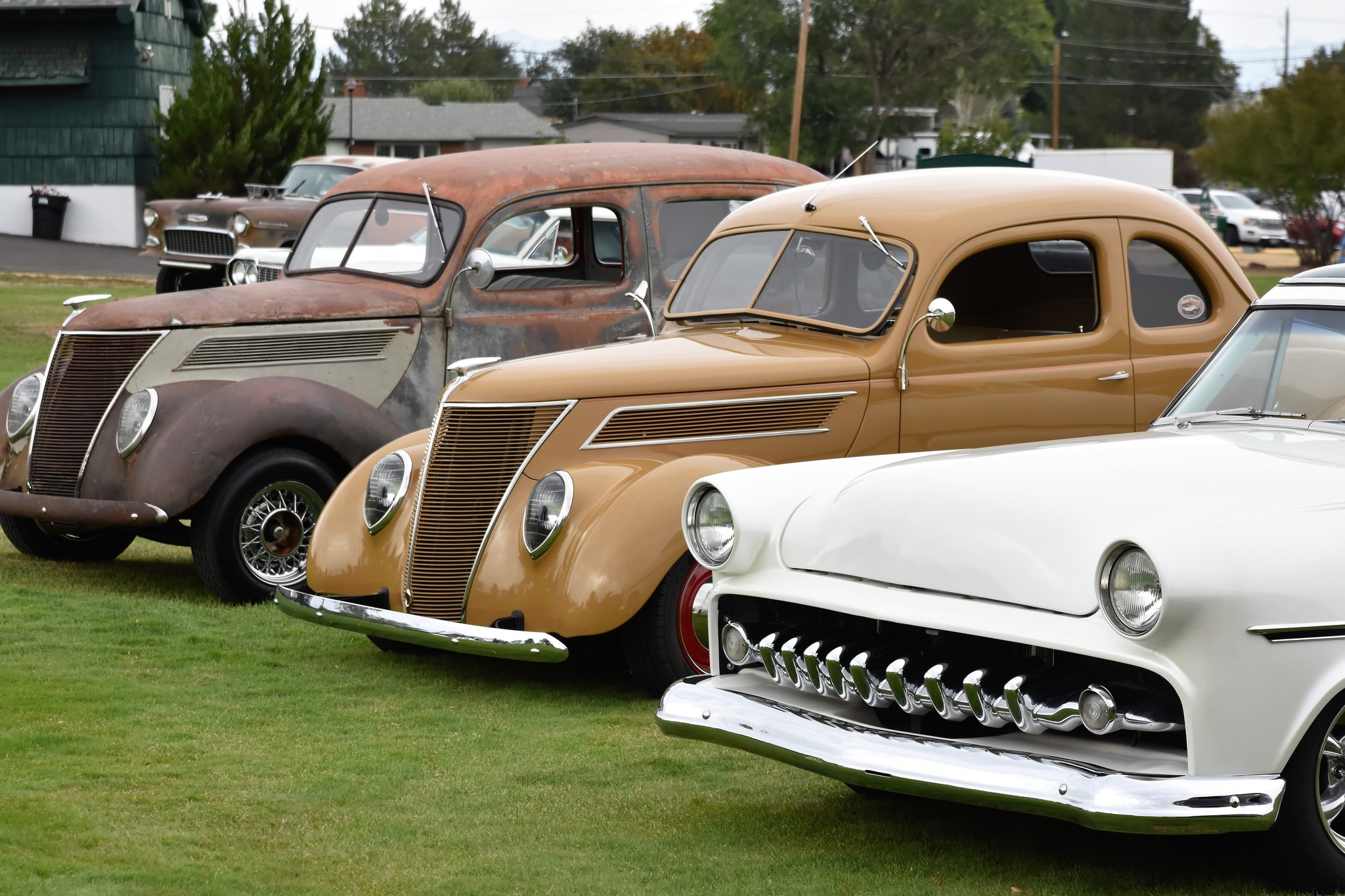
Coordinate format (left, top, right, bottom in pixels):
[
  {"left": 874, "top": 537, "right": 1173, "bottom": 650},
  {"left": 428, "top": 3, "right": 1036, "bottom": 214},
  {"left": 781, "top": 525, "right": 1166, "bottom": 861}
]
[{"left": 29, "top": 192, "right": 71, "bottom": 241}]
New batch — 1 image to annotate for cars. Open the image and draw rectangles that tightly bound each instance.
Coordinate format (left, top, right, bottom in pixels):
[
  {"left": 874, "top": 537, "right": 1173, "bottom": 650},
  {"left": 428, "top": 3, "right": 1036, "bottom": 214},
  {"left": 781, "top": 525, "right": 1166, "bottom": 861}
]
[
  {"left": 1153, "top": 186, "right": 1345, "bottom": 265},
  {"left": 135, "top": 153, "right": 624, "bottom": 295},
  {"left": 652, "top": 260, "right": 1344, "bottom": 896},
  {"left": 0, "top": 142, "right": 835, "bottom": 609},
  {"left": 272, "top": 164, "right": 1344, "bottom": 699}
]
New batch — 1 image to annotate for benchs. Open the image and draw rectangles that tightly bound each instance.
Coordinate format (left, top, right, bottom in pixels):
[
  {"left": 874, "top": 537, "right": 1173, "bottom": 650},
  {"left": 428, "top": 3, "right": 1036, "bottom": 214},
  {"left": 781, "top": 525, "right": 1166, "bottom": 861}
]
[
  {"left": 972, "top": 300, "right": 1093, "bottom": 331},
  {"left": 488, "top": 273, "right": 616, "bottom": 291},
  {"left": 924, "top": 323, "right": 1076, "bottom": 343}
]
